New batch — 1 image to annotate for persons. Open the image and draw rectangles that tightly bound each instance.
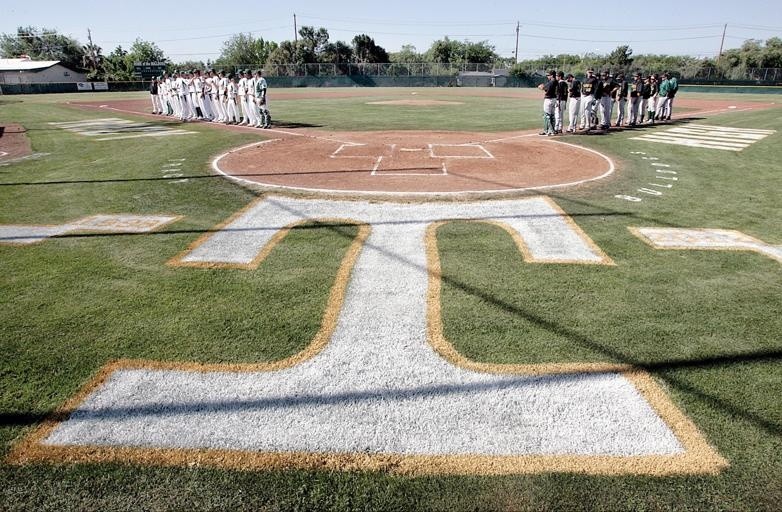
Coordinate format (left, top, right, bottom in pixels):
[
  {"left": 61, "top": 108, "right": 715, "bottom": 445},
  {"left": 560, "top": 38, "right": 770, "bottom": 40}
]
[
  {"left": 537, "top": 68, "right": 679, "bottom": 136},
  {"left": 149, "top": 69, "right": 272, "bottom": 129}
]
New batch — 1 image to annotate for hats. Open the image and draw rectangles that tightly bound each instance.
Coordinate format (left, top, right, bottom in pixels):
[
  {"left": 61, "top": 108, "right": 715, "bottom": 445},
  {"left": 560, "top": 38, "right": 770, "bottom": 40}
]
[
  {"left": 587, "top": 68, "right": 625, "bottom": 79},
  {"left": 151, "top": 69, "right": 233, "bottom": 81},
  {"left": 635, "top": 72, "right": 671, "bottom": 80},
  {"left": 236, "top": 69, "right": 261, "bottom": 75},
  {"left": 545, "top": 70, "right": 572, "bottom": 80}
]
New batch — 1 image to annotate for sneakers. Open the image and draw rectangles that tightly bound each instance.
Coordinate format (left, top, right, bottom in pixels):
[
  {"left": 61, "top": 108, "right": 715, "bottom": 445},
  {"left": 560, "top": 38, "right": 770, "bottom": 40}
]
[
  {"left": 204, "top": 115, "right": 271, "bottom": 129},
  {"left": 615, "top": 116, "right": 671, "bottom": 127},
  {"left": 151, "top": 111, "right": 204, "bottom": 122},
  {"left": 539, "top": 123, "right": 611, "bottom": 135}
]
